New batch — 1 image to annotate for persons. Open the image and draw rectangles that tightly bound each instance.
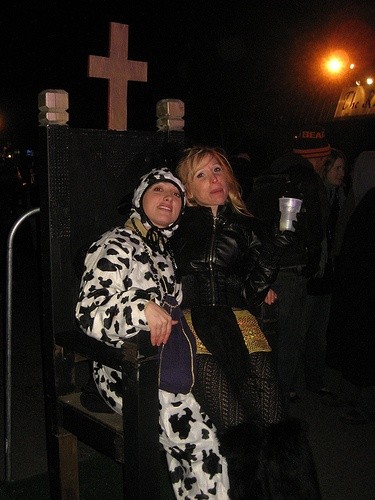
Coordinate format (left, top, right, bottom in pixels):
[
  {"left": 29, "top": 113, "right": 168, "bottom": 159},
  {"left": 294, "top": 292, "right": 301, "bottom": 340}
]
[
  {"left": 176, "top": 146, "right": 324, "bottom": 500},
  {"left": 75, "top": 168, "right": 241, "bottom": 500},
  {"left": 323, "top": 151, "right": 375, "bottom": 426},
  {"left": 245, "top": 126, "right": 332, "bottom": 402},
  {"left": 311, "top": 147, "right": 349, "bottom": 394}
]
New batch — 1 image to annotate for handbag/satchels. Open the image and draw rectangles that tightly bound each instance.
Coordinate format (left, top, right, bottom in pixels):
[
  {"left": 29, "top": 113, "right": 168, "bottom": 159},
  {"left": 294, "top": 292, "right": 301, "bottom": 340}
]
[{"left": 136, "top": 294, "right": 197, "bottom": 394}]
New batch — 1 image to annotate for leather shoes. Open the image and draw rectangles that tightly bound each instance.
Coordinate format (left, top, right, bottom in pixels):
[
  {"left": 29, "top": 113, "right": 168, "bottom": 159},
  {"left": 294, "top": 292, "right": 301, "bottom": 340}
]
[
  {"left": 288, "top": 390, "right": 300, "bottom": 404},
  {"left": 321, "top": 380, "right": 375, "bottom": 424},
  {"left": 312, "top": 390, "right": 335, "bottom": 398}
]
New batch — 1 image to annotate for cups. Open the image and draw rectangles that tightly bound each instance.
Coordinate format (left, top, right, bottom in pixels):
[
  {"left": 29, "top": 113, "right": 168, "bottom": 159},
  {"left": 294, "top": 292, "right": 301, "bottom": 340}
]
[{"left": 279, "top": 197, "right": 303, "bottom": 232}]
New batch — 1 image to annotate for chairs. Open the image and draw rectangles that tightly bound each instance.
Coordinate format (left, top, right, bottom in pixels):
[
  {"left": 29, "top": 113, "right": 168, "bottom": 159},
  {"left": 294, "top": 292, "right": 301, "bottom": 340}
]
[{"left": 38, "top": 88, "right": 285, "bottom": 500}]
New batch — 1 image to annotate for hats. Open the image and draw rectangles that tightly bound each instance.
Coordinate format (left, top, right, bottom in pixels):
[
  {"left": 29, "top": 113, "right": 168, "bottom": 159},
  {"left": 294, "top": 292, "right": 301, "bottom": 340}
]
[{"left": 291, "top": 126, "right": 331, "bottom": 158}]
